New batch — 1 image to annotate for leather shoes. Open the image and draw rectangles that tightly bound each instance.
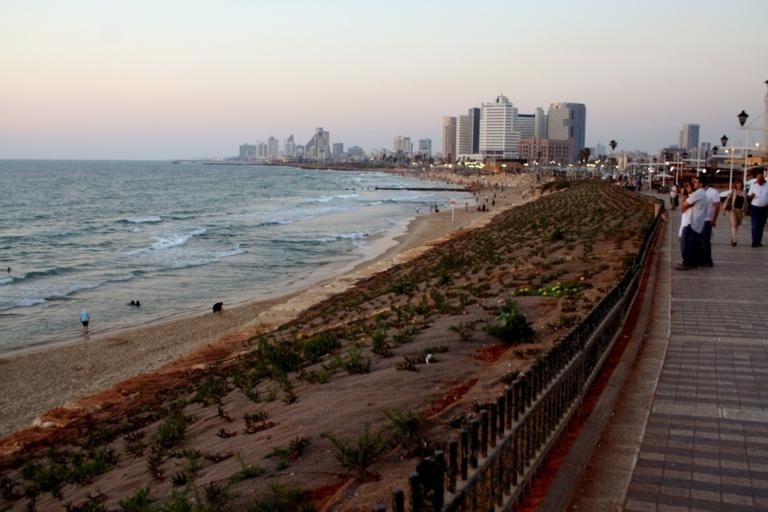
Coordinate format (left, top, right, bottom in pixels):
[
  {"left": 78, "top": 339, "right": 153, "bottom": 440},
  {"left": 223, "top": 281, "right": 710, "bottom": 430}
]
[{"left": 676, "top": 261, "right": 713, "bottom": 270}]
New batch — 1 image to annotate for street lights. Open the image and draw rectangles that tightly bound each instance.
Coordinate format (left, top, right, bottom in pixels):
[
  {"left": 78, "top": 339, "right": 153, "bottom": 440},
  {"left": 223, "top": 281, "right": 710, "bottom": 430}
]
[
  {"left": 737, "top": 110, "right": 750, "bottom": 191},
  {"left": 720, "top": 134, "right": 735, "bottom": 190}
]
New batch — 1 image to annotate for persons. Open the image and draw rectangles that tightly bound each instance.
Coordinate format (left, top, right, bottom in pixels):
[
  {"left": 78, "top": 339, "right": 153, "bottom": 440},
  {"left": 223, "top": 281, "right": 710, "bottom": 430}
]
[
  {"left": 617, "top": 171, "right": 767, "bottom": 270},
  {"left": 79, "top": 307, "right": 90, "bottom": 333},
  {"left": 212, "top": 302, "right": 223, "bottom": 312}
]
